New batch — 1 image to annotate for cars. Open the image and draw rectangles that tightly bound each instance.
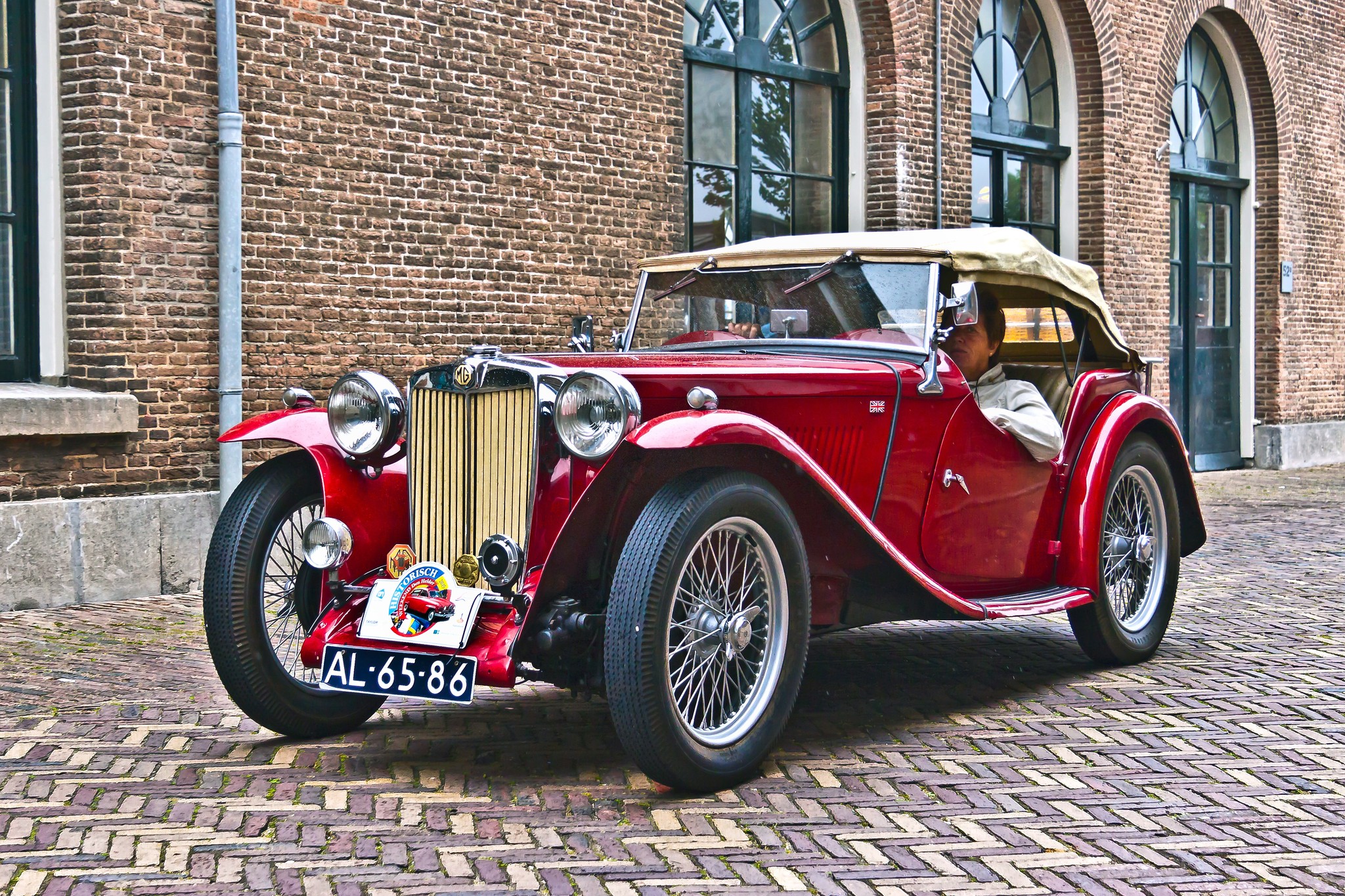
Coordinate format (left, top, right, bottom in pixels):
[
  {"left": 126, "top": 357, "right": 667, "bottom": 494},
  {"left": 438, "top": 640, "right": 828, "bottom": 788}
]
[{"left": 204, "top": 225, "right": 1207, "bottom": 798}]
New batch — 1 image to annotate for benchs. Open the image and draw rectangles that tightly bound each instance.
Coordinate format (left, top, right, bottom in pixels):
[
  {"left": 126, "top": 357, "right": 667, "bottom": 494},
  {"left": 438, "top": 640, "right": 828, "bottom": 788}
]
[{"left": 994, "top": 360, "right": 1078, "bottom": 423}]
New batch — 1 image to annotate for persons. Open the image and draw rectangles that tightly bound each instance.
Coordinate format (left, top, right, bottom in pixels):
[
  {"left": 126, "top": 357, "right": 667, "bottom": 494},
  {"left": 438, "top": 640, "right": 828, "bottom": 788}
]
[
  {"left": 936, "top": 289, "right": 1064, "bottom": 462},
  {"left": 728, "top": 280, "right": 834, "bottom": 339}
]
